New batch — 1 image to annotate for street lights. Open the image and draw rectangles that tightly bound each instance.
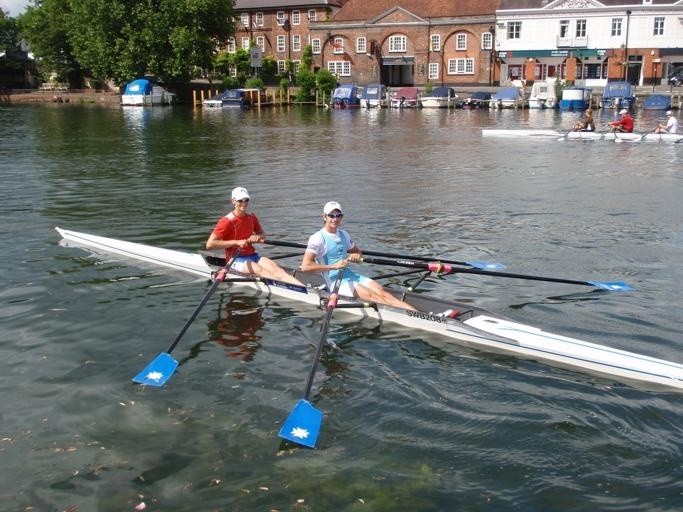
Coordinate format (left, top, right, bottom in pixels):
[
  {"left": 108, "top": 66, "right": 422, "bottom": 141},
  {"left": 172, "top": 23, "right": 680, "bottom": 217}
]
[
  {"left": 488, "top": 26, "right": 497, "bottom": 85},
  {"left": 624, "top": 9, "right": 632, "bottom": 82}
]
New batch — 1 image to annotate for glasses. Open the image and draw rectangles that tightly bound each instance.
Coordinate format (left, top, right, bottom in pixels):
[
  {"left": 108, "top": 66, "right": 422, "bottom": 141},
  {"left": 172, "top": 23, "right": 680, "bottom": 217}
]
[{"left": 328, "top": 212, "right": 343, "bottom": 218}]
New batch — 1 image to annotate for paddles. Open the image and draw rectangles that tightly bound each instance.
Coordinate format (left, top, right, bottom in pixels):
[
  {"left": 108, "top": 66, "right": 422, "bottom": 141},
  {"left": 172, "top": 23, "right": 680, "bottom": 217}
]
[
  {"left": 278, "top": 267, "right": 344, "bottom": 448},
  {"left": 349, "top": 256, "right": 634, "bottom": 292},
  {"left": 132, "top": 248, "right": 241, "bottom": 387},
  {"left": 257, "top": 241, "right": 507, "bottom": 271},
  {"left": 557, "top": 125, "right": 580, "bottom": 141},
  {"left": 631, "top": 128, "right": 657, "bottom": 142},
  {"left": 610, "top": 128, "right": 623, "bottom": 144}
]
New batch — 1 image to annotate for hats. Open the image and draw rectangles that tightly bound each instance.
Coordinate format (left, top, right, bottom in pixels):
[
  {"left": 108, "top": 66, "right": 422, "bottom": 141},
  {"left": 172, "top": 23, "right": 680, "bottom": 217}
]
[
  {"left": 665, "top": 111, "right": 673, "bottom": 116},
  {"left": 323, "top": 201, "right": 343, "bottom": 215},
  {"left": 619, "top": 109, "right": 628, "bottom": 115},
  {"left": 231, "top": 186, "right": 251, "bottom": 201}
]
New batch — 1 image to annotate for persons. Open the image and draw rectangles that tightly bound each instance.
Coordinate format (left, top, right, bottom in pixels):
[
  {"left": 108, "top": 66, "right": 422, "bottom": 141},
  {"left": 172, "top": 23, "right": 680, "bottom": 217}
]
[
  {"left": 300, "top": 201, "right": 453, "bottom": 319},
  {"left": 574, "top": 108, "right": 597, "bottom": 131},
  {"left": 655, "top": 111, "right": 678, "bottom": 134},
  {"left": 206, "top": 186, "right": 327, "bottom": 291},
  {"left": 609, "top": 109, "right": 634, "bottom": 132}
]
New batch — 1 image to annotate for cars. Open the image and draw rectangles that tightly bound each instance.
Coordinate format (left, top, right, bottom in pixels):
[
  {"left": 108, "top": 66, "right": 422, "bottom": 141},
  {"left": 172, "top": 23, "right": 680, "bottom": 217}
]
[{"left": 667, "top": 65, "right": 683, "bottom": 88}]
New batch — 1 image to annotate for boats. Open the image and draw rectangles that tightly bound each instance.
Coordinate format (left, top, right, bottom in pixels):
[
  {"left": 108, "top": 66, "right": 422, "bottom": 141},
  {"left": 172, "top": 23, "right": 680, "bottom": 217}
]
[
  {"left": 203, "top": 88, "right": 248, "bottom": 111},
  {"left": 598, "top": 80, "right": 633, "bottom": 110},
  {"left": 420, "top": 87, "right": 457, "bottom": 110},
  {"left": 121, "top": 79, "right": 177, "bottom": 107},
  {"left": 358, "top": 83, "right": 387, "bottom": 109},
  {"left": 333, "top": 84, "right": 361, "bottom": 110},
  {"left": 528, "top": 80, "right": 559, "bottom": 110},
  {"left": 391, "top": 88, "right": 420, "bottom": 109},
  {"left": 642, "top": 93, "right": 672, "bottom": 110},
  {"left": 487, "top": 85, "right": 524, "bottom": 109},
  {"left": 557, "top": 86, "right": 591, "bottom": 110},
  {"left": 480, "top": 127, "right": 682, "bottom": 148},
  {"left": 52, "top": 225, "right": 682, "bottom": 395},
  {"left": 459, "top": 92, "right": 489, "bottom": 109}
]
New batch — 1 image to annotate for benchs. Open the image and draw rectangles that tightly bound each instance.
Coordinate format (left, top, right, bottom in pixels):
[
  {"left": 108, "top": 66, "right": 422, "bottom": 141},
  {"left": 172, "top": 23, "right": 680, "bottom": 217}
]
[{"left": 38, "top": 83, "right": 69, "bottom": 93}]
